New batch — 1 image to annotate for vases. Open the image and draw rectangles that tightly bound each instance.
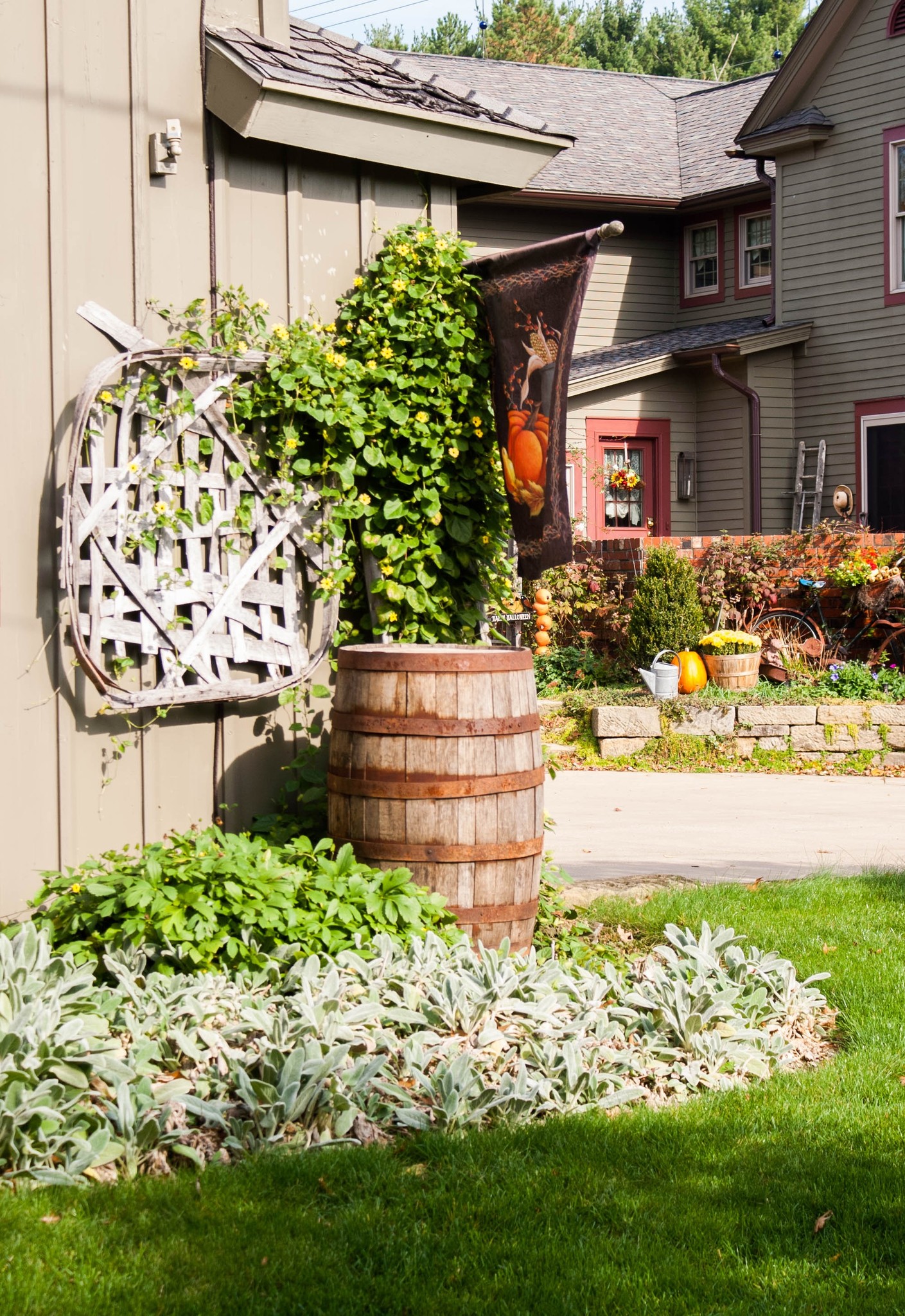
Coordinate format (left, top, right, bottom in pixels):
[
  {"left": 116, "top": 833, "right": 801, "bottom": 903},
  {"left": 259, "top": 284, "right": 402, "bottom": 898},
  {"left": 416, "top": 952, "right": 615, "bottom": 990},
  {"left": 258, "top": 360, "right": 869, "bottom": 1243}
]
[
  {"left": 866, "top": 571, "right": 905, "bottom": 613},
  {"left": 701, "top": 651, "right": 761, "bottom": 692}
]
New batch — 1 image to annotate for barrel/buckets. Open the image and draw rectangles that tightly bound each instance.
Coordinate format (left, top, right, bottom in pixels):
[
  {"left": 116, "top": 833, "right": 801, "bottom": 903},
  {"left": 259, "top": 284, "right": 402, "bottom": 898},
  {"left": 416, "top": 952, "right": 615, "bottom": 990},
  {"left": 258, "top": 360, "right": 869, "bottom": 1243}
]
[
  {"left": 325, "top": 646, "right": 547, "bottom": 956},
  {"left": 700, "top": 652, "right": 763, "bottom": 691}
]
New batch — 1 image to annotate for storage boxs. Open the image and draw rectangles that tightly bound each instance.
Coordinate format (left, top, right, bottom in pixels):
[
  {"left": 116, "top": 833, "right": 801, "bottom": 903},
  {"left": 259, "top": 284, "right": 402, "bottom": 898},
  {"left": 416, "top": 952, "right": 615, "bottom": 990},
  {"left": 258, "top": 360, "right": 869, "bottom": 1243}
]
[{"left": 754, "top": 257, "right": 771, "bottom": 278}]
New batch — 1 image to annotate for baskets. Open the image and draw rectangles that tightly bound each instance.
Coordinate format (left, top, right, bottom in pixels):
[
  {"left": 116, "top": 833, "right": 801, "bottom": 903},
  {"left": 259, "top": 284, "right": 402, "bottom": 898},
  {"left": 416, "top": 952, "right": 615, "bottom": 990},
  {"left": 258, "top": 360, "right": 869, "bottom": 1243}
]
[{"left": 632, "top": 479, "right": 646, "bottom": 490}]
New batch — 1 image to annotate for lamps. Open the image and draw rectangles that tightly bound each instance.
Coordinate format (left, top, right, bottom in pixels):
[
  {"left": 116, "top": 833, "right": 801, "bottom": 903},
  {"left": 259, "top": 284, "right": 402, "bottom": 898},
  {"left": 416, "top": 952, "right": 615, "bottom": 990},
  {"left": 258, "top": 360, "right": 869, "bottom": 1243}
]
[{"left": 677, "top": 451, "right": 695, "bottom": 501}]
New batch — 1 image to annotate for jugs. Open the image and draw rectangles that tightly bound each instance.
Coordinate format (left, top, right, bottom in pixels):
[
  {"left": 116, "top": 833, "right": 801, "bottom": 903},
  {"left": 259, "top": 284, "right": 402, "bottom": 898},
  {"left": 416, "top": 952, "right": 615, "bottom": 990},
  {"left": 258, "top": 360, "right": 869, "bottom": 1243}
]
[{"left": 638, "top": 650, "right": 682, "bottom": 699}]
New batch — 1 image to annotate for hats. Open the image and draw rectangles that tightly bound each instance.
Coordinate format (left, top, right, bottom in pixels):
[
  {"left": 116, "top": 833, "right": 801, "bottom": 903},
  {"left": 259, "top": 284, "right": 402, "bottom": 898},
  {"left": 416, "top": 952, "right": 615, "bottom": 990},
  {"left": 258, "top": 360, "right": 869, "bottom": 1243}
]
[{"left": 833, "top": 485, "right": 853, "bottom": 518}]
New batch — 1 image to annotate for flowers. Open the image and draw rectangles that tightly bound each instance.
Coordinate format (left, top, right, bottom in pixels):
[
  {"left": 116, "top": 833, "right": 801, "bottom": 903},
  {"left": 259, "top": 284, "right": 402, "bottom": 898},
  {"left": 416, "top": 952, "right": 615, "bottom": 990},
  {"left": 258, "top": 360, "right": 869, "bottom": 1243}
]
[
  {"left": 608, "top": 464, "right": 641, "bottom": 492},
  {"left": 828, "top": 546, "right": 878, "bottom": 589},
  {"left": 698, "top": 628, "right": 761, "bottom": 655}
]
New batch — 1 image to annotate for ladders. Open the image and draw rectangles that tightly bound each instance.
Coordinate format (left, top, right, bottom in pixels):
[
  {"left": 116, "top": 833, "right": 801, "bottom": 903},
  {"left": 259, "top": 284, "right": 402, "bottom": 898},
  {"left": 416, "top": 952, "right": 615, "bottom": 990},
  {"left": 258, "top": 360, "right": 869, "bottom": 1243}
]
[{"left": 791, "top": 439, "right": 825, "bottom": 534}]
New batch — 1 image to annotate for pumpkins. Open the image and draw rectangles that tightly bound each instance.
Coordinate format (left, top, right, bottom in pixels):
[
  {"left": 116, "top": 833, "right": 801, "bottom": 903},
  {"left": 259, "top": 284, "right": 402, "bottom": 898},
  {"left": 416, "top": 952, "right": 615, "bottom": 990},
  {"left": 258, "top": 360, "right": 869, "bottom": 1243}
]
[
  {"left": 533, "top": 589, "right": 552, "bottom": 657},
  {"left": 671, "top": 647, "right": 707, "bottom": 694}
]
